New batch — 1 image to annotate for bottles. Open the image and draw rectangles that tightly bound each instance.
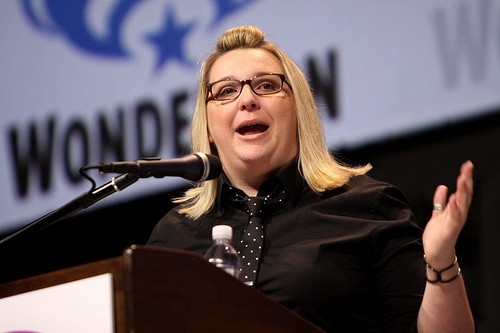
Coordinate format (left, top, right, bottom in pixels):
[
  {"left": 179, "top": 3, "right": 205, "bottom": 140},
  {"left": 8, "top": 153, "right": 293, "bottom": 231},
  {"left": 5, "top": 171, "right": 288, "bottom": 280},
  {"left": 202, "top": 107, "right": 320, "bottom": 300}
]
[{"left": 204, "top": 225, "right": 243, "bottom": 280}]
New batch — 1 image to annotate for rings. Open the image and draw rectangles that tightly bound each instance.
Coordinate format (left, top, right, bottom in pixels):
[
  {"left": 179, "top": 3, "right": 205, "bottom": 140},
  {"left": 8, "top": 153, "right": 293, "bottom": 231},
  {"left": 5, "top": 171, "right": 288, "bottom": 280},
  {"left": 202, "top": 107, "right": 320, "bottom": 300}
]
[{"left": 432, "top": 202, "right": 445, "bottom": 213}]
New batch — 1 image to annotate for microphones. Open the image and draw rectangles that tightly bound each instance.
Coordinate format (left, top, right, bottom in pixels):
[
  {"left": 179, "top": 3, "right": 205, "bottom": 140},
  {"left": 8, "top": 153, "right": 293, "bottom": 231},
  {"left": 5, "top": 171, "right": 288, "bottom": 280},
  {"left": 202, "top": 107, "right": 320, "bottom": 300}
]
[{"left": 98, "top": 152, "right": 223, "bottom": 183}]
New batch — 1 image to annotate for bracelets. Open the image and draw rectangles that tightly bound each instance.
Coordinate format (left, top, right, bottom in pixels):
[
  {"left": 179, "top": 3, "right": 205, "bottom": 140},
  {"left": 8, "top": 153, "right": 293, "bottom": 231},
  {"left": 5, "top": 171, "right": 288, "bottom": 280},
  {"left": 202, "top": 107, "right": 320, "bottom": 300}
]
[{"left": 422, "top": 254, "right": 462, "bottom": 284}]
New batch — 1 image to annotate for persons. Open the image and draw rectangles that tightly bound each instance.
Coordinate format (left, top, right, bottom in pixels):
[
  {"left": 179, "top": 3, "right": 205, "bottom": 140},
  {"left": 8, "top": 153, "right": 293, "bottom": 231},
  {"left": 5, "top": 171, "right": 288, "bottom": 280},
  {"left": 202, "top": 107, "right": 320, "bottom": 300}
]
[{"left": 143, "top": 24, "right": 475, "bottom": 333}]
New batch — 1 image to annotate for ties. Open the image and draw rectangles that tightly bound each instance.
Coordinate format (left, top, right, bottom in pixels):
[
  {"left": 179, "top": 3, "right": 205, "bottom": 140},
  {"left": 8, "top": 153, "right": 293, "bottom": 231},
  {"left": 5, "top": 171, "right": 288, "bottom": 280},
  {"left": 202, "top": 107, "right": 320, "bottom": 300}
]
[{"left": 232, "top": 189, "right": 290, "bottom": 286}]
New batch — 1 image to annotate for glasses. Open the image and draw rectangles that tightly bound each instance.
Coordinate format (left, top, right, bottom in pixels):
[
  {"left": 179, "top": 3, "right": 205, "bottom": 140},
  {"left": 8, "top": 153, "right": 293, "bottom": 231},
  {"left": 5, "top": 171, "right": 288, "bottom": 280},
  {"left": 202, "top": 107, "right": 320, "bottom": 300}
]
[{"left": 204, "top": 72, "right": 292, "bottom": 105}]
